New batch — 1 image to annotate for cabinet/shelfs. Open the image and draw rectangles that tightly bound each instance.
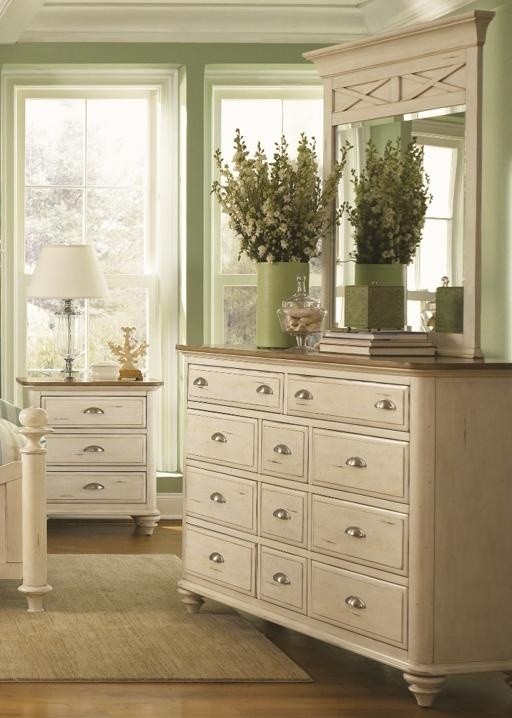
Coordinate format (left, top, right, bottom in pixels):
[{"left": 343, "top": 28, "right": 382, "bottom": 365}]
[
  {"left": 168, "top": 343, "right": 512, "bottom": 704},
  {"left": 14, "top": 367, "right": 166, "bottom": 541}
]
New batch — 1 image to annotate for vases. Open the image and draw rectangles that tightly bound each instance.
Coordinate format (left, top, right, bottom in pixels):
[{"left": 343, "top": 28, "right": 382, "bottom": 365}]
[
  {"left": 350, "top": 251, "right": 412, "bottom": 286},
  {"left": 252, "top": 260, "right": 313, "bottom": 350}
]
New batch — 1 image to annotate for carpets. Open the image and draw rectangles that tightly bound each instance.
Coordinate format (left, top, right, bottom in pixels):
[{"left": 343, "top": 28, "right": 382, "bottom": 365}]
[{"left": 0, "top": 548, "right": 316, "bottom": 689}]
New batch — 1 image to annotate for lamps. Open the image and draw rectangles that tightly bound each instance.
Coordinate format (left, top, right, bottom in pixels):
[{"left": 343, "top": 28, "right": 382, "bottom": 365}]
[{"left": 15, "top": 235, "right": 110, "bottom": 381}]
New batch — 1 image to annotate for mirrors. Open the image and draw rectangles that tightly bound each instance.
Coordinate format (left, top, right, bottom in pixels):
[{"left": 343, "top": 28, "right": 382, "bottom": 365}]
[{"left": 329, "top": 94, "right": 470, "bottom": 343}]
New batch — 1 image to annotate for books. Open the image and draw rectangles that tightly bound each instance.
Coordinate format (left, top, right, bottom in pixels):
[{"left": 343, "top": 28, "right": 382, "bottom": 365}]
[
  {"left": 323, "top": 331, "right": 428, "bottom": 341},
  {"left": 320, "top": 336, "right": 433, "bottom": 347},
  {"left": 319, "top": 343, "right": 437, "bottom": 356}
]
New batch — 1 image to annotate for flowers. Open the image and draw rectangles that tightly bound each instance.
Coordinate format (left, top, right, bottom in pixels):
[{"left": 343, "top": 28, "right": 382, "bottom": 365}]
[
  {"left": 203, "top": 123, "right": 358, "bottom": 262},
  {"left": 348, "top": 125, "right": 436, "bottom": 262}
]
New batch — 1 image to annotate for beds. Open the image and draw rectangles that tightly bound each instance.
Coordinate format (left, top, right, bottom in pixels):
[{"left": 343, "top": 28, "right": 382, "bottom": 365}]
[{"left": 0, "top": 398, "right": 53, "bottom": 612}]
